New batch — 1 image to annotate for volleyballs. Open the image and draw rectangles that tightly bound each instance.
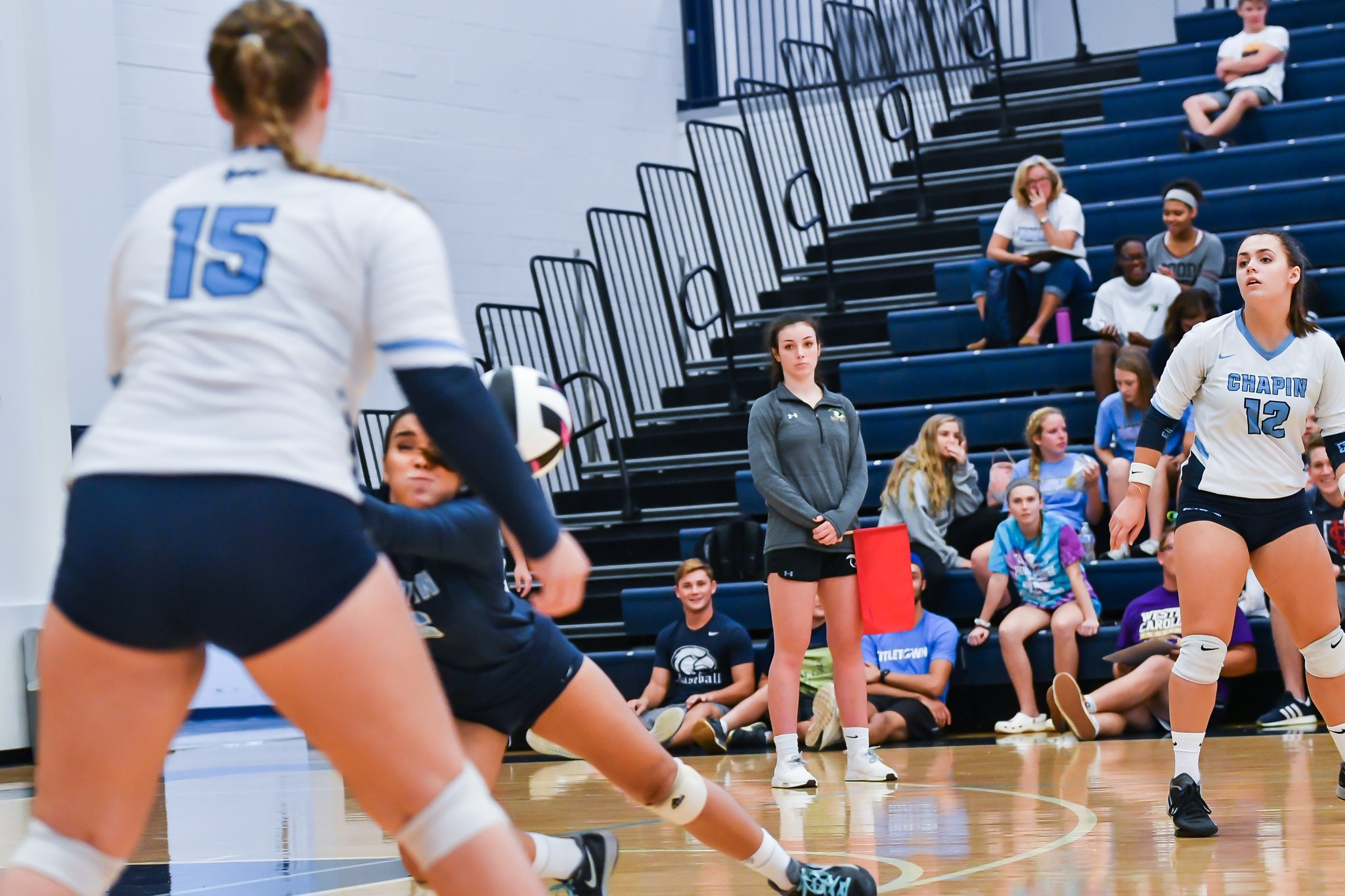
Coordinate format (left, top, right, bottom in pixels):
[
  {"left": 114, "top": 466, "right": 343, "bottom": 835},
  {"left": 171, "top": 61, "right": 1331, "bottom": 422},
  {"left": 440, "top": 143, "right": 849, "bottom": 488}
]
[{"left": 480, "top": 365, "right": 573, "bottom": 480}]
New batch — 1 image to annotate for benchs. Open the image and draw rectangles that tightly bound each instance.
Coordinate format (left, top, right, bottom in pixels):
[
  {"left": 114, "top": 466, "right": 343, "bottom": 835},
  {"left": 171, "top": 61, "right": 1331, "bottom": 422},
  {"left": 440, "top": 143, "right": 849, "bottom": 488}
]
[{"left": 504, "top": 0, "right": 1345, "bottom": 733}]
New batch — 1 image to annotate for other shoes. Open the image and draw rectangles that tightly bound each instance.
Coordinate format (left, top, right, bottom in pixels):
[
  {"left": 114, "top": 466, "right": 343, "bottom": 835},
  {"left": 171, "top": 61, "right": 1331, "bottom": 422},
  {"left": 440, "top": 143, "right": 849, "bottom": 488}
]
[
  {"left": 1046, "top": 672, "right": 1099, "bottom": 741},
  {"left": 1020, "top": 337, "right": 1036, "bottom": 345},
  {"left": 1139, "top": 539, "right": 1159, "bottom": 554},
  {"left": 1180, "top": 128, "right": 1212, "bottom": 152},
  {"left": 1109, "top": 544, "right": 1132, "bottom": 560},
  {"left": 692, "top": 715, "right": 728, "bottom": 753},
  {"left": 648, "top": 708, "right": 685, "bottom": 744},
  {"left": 525, "top": 729, "right": 583, "bottom": 760},
  {"left": 804, "top": 682, "right": 840, "bottom": 752},
  {"left": 996, "top": 712, "right": 1046, "bottom": 734},
  {"left": 968, "top": 342, "right": 981, "bottom": 349},
  {"left": 726, "top": 723, "right": 766, "bottom": 747}
]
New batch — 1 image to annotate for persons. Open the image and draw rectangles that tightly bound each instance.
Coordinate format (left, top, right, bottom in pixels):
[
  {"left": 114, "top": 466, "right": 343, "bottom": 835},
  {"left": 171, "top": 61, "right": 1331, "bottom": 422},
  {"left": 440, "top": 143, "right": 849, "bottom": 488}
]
[
  {"left": 1181, "top": 0, "right": 1291, "bottom": 151},
  {"left": 1, "top": 0, "right": 592, "bottom": 896},
  {"left": 350, "top": 154, "right": 1345, "bottom": 896}
]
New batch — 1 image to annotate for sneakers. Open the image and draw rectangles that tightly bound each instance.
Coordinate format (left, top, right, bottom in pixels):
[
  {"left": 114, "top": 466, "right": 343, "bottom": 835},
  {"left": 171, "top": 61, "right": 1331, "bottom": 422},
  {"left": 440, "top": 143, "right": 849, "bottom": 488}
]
[
  {"left": 768, "top": 857, "right": 876, "bottom": 895},
  {"left": 1167, "top": 772, "right": 1218, "bottom": 835},
  {"left": 1257, "top": 689, "right": 1318, "bottom": 726},
  {"left": 843, "top": 745, "right": 898, "bottom": 782},
  {"left": 771, "top": 751, "right": 818, "bottom": 788},
  {"left": 1335, "top": 762, "right": 1345, "bottom": 800},
  {"left": 551, "top": 830, "right": 620, "bottom": 896}
]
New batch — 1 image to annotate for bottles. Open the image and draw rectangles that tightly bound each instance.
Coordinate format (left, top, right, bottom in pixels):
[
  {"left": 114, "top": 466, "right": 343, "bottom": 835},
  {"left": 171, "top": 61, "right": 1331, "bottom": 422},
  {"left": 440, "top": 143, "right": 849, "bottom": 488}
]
[
  {"left": 1079, "top": 522, "right": 1095, "bottom": 563},
  {"left": 1055, "top": 306, "right": 1072, "bottom": 343}
]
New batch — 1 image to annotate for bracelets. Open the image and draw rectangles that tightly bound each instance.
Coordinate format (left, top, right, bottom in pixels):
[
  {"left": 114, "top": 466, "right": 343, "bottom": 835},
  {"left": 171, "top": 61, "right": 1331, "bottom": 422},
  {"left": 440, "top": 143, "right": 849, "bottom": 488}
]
[
  {"left": 974, "top": 618, "right": 992, "bottom": 628},
  {"left": 1129, "top": 462, "right": 1155, "bottom": 487}
]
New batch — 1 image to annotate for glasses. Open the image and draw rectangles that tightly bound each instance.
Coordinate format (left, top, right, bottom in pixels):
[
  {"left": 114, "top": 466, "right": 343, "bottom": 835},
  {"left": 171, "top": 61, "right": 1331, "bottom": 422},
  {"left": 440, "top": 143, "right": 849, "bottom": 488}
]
[
  {"left": 1028, "top": 176, "right": 1049, "bottom": 185},
  {"left": 1121, "top": 254, "right": 1142, "bottom": 260},
  {"left": 1161, "top": 543, "right": 1173, "bottom": 551}
]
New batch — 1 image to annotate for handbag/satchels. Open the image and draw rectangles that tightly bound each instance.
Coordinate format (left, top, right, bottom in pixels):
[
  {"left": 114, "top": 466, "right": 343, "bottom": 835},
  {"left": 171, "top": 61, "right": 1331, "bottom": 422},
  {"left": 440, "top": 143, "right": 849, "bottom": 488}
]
[{"left": 986, "top": 448, "right": 1016, "bottom": 507}]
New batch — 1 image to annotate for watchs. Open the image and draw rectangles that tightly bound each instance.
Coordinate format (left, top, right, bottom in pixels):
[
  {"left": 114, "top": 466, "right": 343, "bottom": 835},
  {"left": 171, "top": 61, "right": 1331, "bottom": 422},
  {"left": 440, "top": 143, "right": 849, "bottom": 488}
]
[
  {"left": 880, "top": 669, "right": 890, "bottom": 684},
  {"left": 1040, "top": 217, "right": 1049, "bottom": 226}
]
